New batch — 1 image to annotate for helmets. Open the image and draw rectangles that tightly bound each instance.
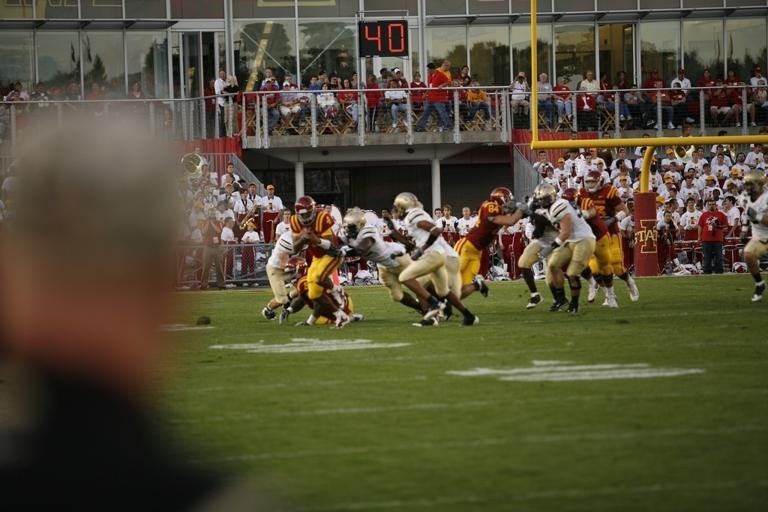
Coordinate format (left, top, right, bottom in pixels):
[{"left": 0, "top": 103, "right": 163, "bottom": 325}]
[
  {"left": 393, "top": 192, "right": 418, "bottom": 220},
  {"left": 295, "top": 196, "right": 317, "bottom": 226},
  {"left": 284, "top": 256, "right": 307, "bottom": 282},
  {"left": 532, "top": 182, "right": 556, "bottom": 207},
  {"left": 490, "top": 187, "right": 515, "bottom": 214},
  {"left": 741, "top": 171, "right": 766, "bottom": 196},
  {"left": 341, "top": 210, "right": 366, "bottom": 241}
]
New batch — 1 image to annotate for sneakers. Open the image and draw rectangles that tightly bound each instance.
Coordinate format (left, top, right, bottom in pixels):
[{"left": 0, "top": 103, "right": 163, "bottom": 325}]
[
  {"left": 526, "top": 292, "right": 544, "bottom": 309},
  {"left": 601, "top": 288, "right": 619, "bottom": 309},
  {"left": 295, "top": 321, "right": 313, "bottom": 327},
  {"left": 475, "top": 274, "right": 489, "bottom": 298},
  {"left": 330, "top": 311, "right": 363, "bottom": 330},
  {"left": 261, "top": 292, "right": 293, "bottom": 324},
  {"left": 548, "top": 296, "right": 569, "bottom": 312},
  {"left": 586, "top": 281, "right": 600, "bottom": 303},
  {"left": 412, "top": 297, "right": 453, "bottom": 327},
  {"left": 750, "top": 281, "right": 766, "bottom": 301},
  {"left": 459, "top": 314, "right": 480, "bottom": 326},
  {"left": 566, "top": 295, "right": 579, "bottom": 313},
  {"left": 628, "top": 277, "right": 640, "bottom": 303}
]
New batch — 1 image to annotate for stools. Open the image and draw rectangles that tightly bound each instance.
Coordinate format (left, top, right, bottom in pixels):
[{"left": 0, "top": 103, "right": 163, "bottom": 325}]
[
  {"left": 232, "top": 106, "right": 530, "bottom": 136},
  {"left": 537, "top": 107, "right": 624, "bottom": 135}
]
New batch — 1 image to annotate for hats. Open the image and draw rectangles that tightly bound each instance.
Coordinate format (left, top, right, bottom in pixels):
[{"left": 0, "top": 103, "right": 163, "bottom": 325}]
[
  {"left": 559, "top": 146, "right": 739, "bottom": 204},
  {"left": 193, "top": 181, "right": 275, "bottom": 229}
]
